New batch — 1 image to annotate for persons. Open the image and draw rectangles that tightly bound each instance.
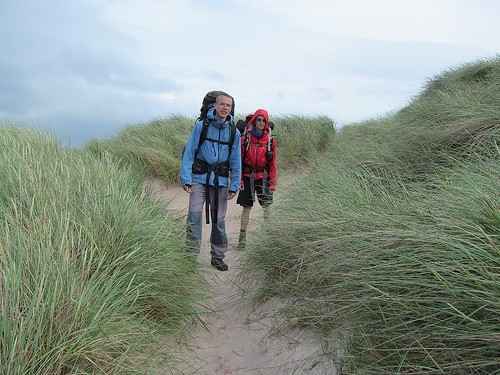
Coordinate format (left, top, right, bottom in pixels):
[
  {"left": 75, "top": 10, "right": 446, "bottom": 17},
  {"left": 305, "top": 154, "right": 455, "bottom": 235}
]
[
  {"left": 237, "top": 109, "right": 278, "bottom": 251},
  {"left": 178, "top": 95, "right": 242, "bottom": 270}
]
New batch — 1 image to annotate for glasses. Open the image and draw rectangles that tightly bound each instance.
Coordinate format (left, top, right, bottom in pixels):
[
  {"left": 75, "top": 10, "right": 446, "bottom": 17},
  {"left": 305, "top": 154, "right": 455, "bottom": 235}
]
[
  {"left": 255, "top": 118, "right": 266, "bottom": 123},
  {"left": 217, "top": 103, "right": 233, "bottom": 107}
]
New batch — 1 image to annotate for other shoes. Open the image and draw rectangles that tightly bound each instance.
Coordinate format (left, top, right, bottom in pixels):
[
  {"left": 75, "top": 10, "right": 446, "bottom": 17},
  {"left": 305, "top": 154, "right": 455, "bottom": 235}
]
[
  {"left": 211, "top": 257, "right": 228, "bottom": 271},
  {"left": 238, "top": 233, "right": 247, "bottom": 250}
]
